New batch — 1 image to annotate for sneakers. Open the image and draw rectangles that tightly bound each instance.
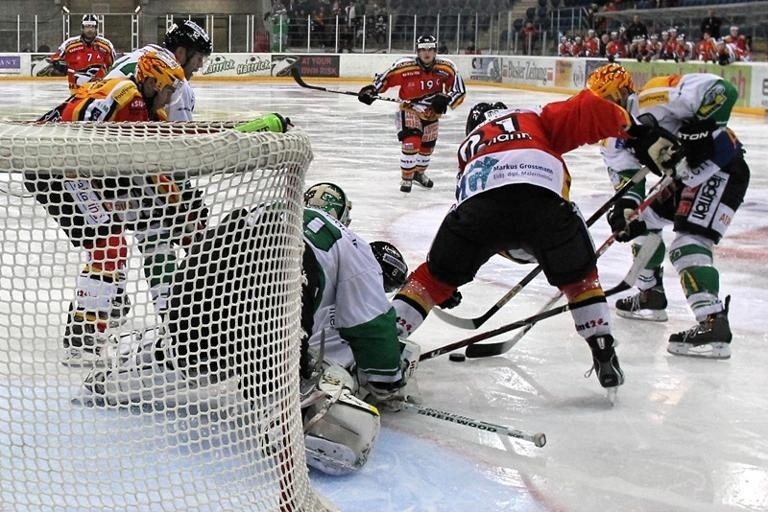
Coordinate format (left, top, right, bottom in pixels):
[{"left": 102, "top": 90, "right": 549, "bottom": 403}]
[
  {"left": 400, "top": 178, "right": 412, "bottom": 192},
  {"left": 587, "top": 333, "right": 623, "bottom": 387},
  {"left": 670, "top": 312, "right": 732, "bottom": 345},
  {"left": 413, "top": 171, "right": 433, "bottom": 187},
  {"left": 616, "top": 285, "right": 666, "bottom": 311},
  {"left": 63, "top": 331, "right": 108, "bottom": 356}
]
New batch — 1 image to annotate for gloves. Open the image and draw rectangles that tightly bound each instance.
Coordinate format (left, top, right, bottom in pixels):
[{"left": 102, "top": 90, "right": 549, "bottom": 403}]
[
  {"left": 424, "top": 92, "right": 451, "bottom": 114},
  {"left": 608, "top": 119, "right": 715, "bottom": 242},
  {"left": 358, "top": 86, "right": 379, "bottom": 104}
]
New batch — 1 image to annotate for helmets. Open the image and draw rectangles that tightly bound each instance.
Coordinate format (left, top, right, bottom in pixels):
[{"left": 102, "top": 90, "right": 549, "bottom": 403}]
[
  {"left": 135, "top": 51, "right": 184, "bottom": 93},
  {"left": 165, "top": 20, "right": 212, "bottom": 53},
  {"left": 303, "top": 183, "right": 352, "bottom": 227},
  {"left": 466, "top": 102, "right": 508, "bottom": 136},
  {"left": 81, "top": 14, "right": 98, "bottom": 29},
  {"left": 369, "top": 241, "right": 408, "bottom": 293},
  {"left": 417, "top": 35, "right": 438, "bottom": 49},
  {"left": 587, "top": 64, "right": 635, "bottom": 108}
]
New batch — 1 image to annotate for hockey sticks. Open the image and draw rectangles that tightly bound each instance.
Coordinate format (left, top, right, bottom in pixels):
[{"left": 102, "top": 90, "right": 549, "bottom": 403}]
[
  {"left": 420, "top": 231, "right": 660, "bottom": 363},
  {"left": 430, "top": 149, "right": 672, "bottom": 329},
  {"left": 292, "top": 67, "right": 431, "bottom": 105},
  {"left": 465, "top": 176, "right": 672, "bottom": 358}
]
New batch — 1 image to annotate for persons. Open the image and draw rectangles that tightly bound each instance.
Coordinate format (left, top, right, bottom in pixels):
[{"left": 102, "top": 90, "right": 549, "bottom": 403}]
[
  {"left": 389, "top": 87, "right": 624, "bottom": 407},
  {"left": 358, "top": 34, "right": 481, "bottom": 193},
  {"left": 587, "top": 61, "right": 750, "bottom": 357},
  {"left": 519, "top": 23, "right": 537, "bottom": 55},
  {"left": 557, "top": 2, "right": 751, "bottom": 66},
  {"left": 21, "top": 0, "right": 421, "bottom": 478}
]
